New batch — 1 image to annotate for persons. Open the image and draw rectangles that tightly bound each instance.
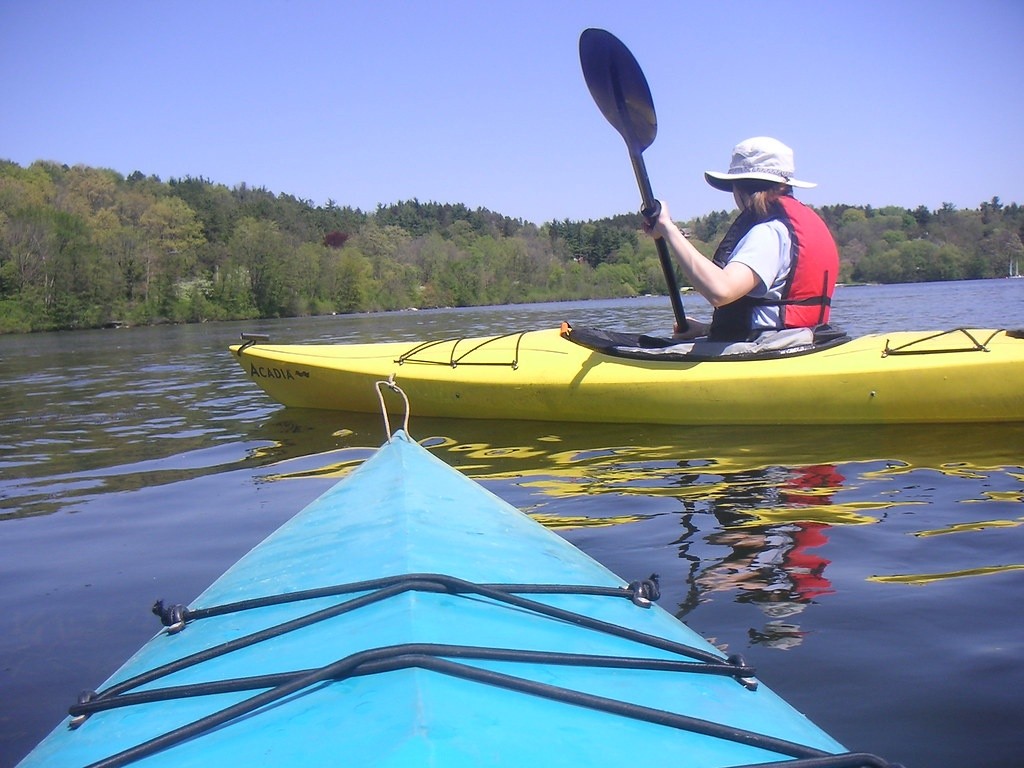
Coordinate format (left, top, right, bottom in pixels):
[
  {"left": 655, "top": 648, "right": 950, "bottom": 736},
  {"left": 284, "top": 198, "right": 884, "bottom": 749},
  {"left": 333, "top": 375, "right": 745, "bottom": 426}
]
[{"left": 641, "top": 137, "right": 839, "bottom": 343}]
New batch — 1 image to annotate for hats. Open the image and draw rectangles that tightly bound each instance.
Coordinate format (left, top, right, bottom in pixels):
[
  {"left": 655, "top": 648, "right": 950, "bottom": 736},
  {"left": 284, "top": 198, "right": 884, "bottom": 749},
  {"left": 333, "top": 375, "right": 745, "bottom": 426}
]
[{"left": 703, "top": 136, "right": 818, "bottom": 193}]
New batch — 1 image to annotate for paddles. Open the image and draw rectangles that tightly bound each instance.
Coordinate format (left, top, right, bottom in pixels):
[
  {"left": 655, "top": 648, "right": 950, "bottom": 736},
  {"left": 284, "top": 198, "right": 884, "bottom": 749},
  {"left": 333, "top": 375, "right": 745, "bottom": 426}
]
[{"left": 577, "top": 26, "right": 691, "bottom": 337}]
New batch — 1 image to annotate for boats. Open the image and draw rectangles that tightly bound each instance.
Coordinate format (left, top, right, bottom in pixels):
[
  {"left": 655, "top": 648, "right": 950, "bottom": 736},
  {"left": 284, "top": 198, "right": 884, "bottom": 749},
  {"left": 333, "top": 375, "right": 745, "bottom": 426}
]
[
  {"left": 11, "top": 430, "right": 890, "bottom": 767},
  {"left": 229, "top": 320, "right": 1024, "bottom": 427}
]
